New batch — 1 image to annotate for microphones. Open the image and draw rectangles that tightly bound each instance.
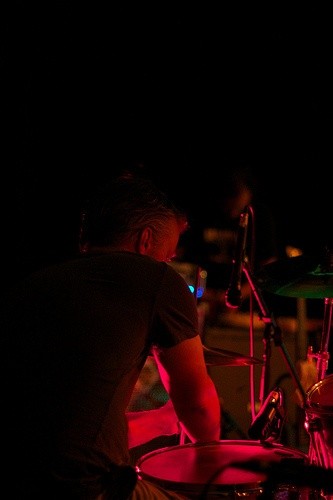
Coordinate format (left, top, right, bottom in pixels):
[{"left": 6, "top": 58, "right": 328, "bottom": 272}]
[
  {"left": 248, "top": 388, "right": 280, "bottom": 439},
  {"left": 225, "top": 208, "right": 249, "bottom": 308}
]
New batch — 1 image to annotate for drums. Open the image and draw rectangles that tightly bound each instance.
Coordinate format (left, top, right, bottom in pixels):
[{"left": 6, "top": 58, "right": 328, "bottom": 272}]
[
  {"left": 306, "top": 375, "right": 333, "bottom": 448},
  {"left": 136, "top": 440, "right": 309, "bottom": 500}
]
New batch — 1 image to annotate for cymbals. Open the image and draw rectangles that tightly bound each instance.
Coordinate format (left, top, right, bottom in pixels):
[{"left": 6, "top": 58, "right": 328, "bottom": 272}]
[{"left": 275, "top": 271, "right": 333, "bottom": 299}]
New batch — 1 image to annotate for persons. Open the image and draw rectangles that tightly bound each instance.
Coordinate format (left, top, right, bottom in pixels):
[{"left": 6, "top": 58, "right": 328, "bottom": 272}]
[
  {"left": 0, "top": 168, "right": 222, "bottom": 500},
  {"left": 185, "top": 167, "right": 253, "bottom": 310}
]
